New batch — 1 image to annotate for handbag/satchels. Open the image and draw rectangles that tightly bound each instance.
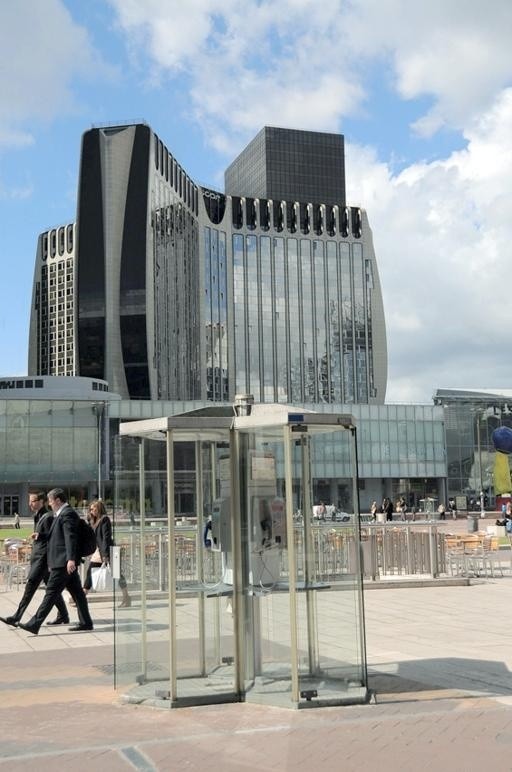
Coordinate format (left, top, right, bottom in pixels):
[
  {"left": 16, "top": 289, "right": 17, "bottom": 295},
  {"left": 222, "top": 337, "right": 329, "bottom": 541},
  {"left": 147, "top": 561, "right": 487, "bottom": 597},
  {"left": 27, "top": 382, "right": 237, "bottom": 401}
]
[{"left": 91, "top": 562, "right": 118, "bottom": 592}]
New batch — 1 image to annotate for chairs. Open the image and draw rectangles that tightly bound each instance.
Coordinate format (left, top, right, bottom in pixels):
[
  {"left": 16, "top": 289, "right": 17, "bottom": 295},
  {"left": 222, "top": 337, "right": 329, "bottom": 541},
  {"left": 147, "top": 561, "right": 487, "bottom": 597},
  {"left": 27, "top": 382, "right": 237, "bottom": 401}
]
[{"left": 0, "top": 527, "right": 512, "bottom": 592}]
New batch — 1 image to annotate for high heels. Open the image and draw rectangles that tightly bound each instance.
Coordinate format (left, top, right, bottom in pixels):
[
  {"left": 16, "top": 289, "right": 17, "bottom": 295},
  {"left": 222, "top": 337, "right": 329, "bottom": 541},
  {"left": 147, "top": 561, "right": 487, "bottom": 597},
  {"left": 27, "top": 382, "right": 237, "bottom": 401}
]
[{"left": 118, "top": 598, "right": 131, "bottom": 608}]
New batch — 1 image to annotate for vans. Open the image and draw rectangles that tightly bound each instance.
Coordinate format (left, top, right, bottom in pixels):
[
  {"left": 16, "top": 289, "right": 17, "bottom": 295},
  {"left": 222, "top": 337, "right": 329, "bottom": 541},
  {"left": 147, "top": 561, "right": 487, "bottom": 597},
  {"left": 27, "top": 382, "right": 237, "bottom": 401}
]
[{"left": 312, "top": 505, "right": 350, "bottom": 523}]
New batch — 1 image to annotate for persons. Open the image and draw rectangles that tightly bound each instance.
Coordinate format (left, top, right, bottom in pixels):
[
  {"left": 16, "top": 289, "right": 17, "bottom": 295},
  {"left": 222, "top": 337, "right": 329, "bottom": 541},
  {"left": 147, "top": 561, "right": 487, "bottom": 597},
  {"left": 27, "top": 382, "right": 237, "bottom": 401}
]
[
  {"left": 502, "top": 513, "right": 512, "bottom": 538},
  {"left": 314, "top": 501, "right": 327, "bottom": 523},
  {"left": 330, "top": 502, "right": 340, "bottom": 522},
  {"left": 1, "top": 489, "right": 71, "bottom": 627},
  {"left": 501, "top": 503, "right": 508, "bottom": 519},
  {"left": 13, "top": 486, "right": 95, "bottom": 635},
  {"left": 380, "top": 497, "right": 387, "bottom": 513},
  {"left": 447, "top": 496, "right": 458, "bottom": 520},
  {"left": 437, "top": 504, "right": 446, "bottom": 520},
  {"left": 383, "top": 496, "right": 394, "bottom": 522},
  {"left": 399, "top": 496, "right": 408, "bottom": 522},
  {"left": 367, "top": 501, "right": 378, "bottom": 524},
  {"left": 13, "top": 512, "right": 21, "bottom": 529},
  {"left": 66, "top": 500, "right": 132, "bottom": 608},
  {"left": 506, "top": 500, "right": 512, "bottom": 520}
]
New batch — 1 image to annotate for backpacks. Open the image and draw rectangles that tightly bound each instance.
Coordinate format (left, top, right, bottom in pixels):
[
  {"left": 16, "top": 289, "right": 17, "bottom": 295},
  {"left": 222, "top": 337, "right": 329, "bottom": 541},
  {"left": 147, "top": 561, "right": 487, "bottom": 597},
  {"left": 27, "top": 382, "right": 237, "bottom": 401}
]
[{"left": 77, "top": 518, "right": 96, "bottom": 558}]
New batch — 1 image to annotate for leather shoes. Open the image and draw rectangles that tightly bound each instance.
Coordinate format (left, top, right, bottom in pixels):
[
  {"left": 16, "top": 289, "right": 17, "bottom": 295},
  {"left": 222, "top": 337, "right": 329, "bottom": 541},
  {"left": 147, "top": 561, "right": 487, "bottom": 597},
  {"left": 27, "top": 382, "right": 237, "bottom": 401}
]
[
  {"left": 16, "top": 622, "right": 38, "bottom": 634},
  {"left": 47, "top": 617, "right": 69, "bottom": 625},
  {"left": 68, "top": 624, "right": 93, "bottom": 631},
  {"left": 0, "top": 617, "right": 20, "bottom": 627}
]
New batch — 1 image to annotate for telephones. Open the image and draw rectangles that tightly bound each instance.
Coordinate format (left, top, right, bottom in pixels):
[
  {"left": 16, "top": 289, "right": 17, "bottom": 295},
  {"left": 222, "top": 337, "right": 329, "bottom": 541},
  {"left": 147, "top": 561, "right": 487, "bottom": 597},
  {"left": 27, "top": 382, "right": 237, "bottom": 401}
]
[
  {"left": 204, "top": 498, "right": 229, "bottom": 554},
  {"left": 256, "top": 499, "right": 286, "bottom": 551}
]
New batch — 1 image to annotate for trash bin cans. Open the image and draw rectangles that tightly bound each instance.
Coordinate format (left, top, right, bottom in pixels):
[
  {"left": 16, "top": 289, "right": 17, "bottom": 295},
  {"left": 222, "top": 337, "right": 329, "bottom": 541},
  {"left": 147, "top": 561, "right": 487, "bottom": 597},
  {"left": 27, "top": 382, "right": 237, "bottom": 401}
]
[
  {"left": 467, "top": 515, "right": 477, "bottom": 532},
  {"left": 350, "top": 536, "right": 379, "bottom": 580}
]
